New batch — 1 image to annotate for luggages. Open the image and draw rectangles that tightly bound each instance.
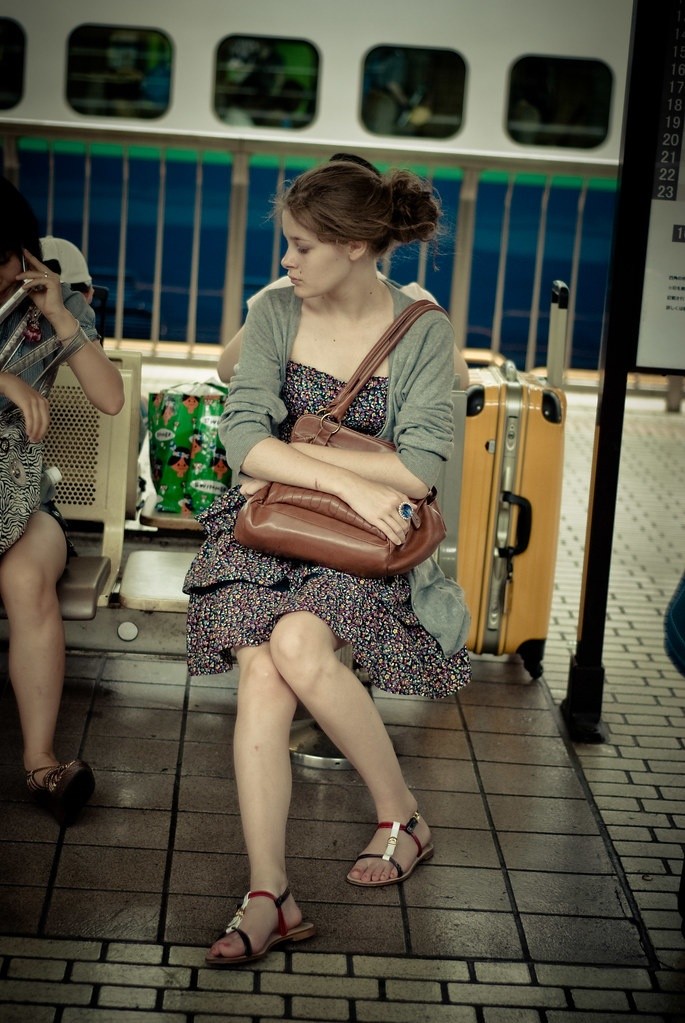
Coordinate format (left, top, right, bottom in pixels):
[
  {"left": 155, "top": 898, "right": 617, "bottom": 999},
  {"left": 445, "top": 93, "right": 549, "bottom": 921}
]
[{"left": 454, "top": 358, "right": 567, "bottom": 680}]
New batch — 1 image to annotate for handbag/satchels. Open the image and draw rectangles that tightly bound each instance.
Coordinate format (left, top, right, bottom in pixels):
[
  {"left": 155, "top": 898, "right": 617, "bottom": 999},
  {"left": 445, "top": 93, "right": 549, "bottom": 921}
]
[
  {"left": 148, "top": 378, "right": 231, "bottom": 520},
  {"left": 235, "top": 300, "right": 452, "bottom": 579},
  {"left": 1, "top": 277, "right": 90, "bottom": 557}
]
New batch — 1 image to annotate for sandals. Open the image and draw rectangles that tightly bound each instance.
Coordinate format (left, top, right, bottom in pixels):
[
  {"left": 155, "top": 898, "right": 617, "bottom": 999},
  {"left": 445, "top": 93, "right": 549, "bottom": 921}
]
[
  {"left": 27, "top": 759, "right": 96, "bottom": 826},
  {"left": 346, "top": 807, "right": 435, "bottom": 888},
  {"left": 205, "top": 886, "right": 317, "bottom": 965}
]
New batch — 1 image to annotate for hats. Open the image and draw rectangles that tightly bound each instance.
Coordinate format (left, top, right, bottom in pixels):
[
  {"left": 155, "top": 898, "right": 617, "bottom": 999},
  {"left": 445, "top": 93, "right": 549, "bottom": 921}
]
[{"left": 38, "top": 235, "right": 92, "bottom": 285}]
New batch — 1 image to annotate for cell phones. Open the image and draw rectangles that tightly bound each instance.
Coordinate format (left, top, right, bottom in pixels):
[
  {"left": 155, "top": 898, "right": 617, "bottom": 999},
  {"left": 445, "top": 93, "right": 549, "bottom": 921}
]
[{"left": 23, "top": 254, "right": 43, "bottom": 292}]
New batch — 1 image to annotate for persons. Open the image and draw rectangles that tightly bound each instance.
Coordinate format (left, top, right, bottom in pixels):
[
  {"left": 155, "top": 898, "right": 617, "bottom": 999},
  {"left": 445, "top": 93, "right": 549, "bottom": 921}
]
[
  {"left": 36, "top": 234, "right": 96, "bottom": 306},
  {"left": 0, "top": 210, "right": 129, "bottom": 814},
  {"left": 182, "top": 149, "right": 472, "bottom": 969}
]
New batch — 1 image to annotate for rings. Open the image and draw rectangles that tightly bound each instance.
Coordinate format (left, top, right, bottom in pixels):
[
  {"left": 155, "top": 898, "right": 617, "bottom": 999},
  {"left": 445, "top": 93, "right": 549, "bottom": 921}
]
[
  {"left": 398, "top": 500, "right": 414, "bottom": 520},
  {"left": 44, "top": 270, "right": 48, "bottom": 277}
]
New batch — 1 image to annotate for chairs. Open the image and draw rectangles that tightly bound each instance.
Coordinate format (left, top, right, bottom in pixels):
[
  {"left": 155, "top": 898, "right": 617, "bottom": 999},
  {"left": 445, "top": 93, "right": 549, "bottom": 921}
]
[
  {"left": 62, "top": 350, "right": 151, "bottom": 521},
  {"left": 0, "top": 366, "right": 134, "bottom": 620},
  {"left": 121, "top": 390, "right": 469, "bottom": 613}
]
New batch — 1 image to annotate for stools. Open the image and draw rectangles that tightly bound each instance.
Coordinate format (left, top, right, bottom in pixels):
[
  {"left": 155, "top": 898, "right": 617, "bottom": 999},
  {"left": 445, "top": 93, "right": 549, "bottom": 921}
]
[{"left": 138, "top": 491, "right": 205, "bottom": 533}]
